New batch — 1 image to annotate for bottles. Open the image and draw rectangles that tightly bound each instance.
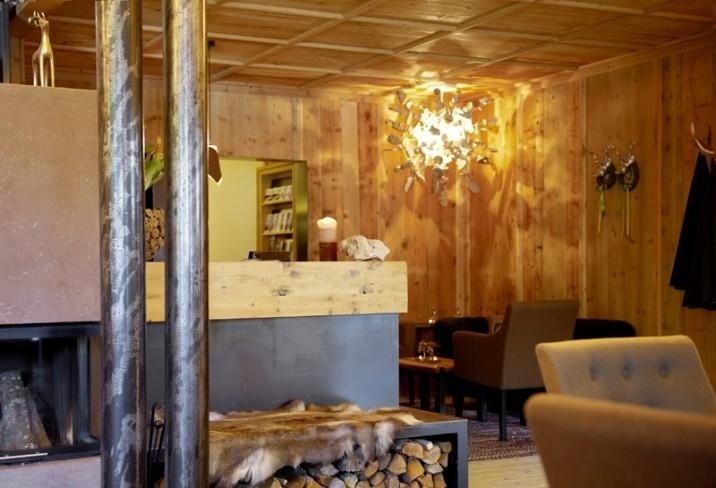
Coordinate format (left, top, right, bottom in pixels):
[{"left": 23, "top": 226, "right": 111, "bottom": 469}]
[
  {"left": 428, "top": 310, "right": 439, "bottom": 325},
  {"left": 454, "top": 309, "right": 463, "bottom": 317}
]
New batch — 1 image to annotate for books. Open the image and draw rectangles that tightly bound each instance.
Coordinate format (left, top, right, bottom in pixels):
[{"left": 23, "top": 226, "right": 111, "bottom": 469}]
[{"left": 263, "top": 185, "right": 294, "bottom": 253}]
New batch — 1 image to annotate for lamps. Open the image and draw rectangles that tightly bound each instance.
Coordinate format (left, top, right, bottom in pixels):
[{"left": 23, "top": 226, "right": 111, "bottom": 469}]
[{"left": 385, "top": 88, "right": 498, "bottom": 207}]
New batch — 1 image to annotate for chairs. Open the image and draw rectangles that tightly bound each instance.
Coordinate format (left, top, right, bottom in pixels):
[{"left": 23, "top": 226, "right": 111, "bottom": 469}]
[
  {"left": 399, "top": 297, "right": 581, "bottom": 441},
  {"left": 523, "top": 335, "right": 716, "bottom": 487}
]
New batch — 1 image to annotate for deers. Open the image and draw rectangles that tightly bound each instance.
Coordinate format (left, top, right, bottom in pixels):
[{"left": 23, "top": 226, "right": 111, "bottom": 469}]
[{"left": 27, "top": 10, "right": 56, "bottom": 87}]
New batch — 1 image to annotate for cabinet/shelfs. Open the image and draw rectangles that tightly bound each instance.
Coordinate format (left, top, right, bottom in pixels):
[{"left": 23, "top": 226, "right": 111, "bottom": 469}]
[{"left": 256, "top": 161, "right": 308, "bottom": 262}]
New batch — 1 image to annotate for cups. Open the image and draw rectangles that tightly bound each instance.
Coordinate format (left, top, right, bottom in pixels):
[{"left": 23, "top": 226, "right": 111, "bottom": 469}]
[{"left": 317, "top": 217, "right": 338, "bottom": 261}]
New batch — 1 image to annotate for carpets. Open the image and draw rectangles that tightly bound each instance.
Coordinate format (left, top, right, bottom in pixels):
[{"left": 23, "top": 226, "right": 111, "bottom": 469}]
[{"left": 400, "top": 400, "right": 537, "bottom": 462}]
